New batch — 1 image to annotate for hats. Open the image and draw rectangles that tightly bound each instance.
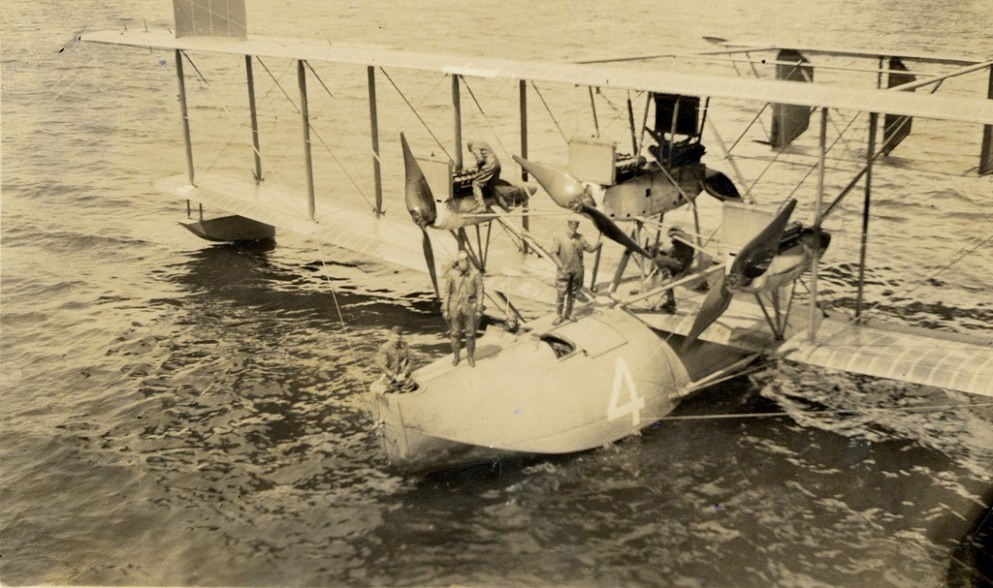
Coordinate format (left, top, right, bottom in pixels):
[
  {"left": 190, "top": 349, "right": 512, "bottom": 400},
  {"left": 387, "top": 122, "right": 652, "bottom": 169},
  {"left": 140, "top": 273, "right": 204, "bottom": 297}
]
[{"left": 567, "top": 220, "right": 580, "bottom": 224}]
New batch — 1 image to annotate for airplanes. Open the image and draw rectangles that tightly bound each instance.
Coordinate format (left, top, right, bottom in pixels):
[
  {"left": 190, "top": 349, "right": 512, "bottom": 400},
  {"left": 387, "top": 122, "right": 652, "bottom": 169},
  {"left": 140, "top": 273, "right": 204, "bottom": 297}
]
[{"left": 81, "top": 1, "right": 992, "bottom": 476}]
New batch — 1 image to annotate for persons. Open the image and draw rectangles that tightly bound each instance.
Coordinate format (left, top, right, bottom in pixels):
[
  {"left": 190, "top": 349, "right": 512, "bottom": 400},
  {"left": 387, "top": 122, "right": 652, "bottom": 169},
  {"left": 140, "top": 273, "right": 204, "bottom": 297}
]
[
  {"left": 655, "top": 226, "right": 693, "bottom": 309},
  {"left": 440, "top": 251, "right": 485, "bottom": 367},
  {"left": 467, "top": 139, "right": 508, "bottom": 215},
  {"left": 376, "top": 326, "right": 417, "bottom": 392},
  {"left": 552, "top": 219, "right": 603, "bottom": 325}
]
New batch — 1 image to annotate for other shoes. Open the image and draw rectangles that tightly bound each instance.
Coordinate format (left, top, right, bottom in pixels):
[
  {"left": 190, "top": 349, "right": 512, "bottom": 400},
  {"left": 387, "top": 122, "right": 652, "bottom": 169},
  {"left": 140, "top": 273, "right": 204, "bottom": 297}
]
[
  {"left": 452, "top": 354, "right": 459, "bottom": 366},
  {"left": 467, "top": 353, "right": 475, "bottom": 367},
  {"left": 553, "top": 313, "right": 563, "bottom": 325},
  {"left": 662, "top": 298, "right": 675, "bottom": 308},
  {"left": 471, "top": 200, "right": 487, "bottom": 213},
  {"left": 565, "top": 311, "right": 577, "bottom": 322},
  {"left": 660, "top": 268, "right": 673, "bottom": 285}
]
